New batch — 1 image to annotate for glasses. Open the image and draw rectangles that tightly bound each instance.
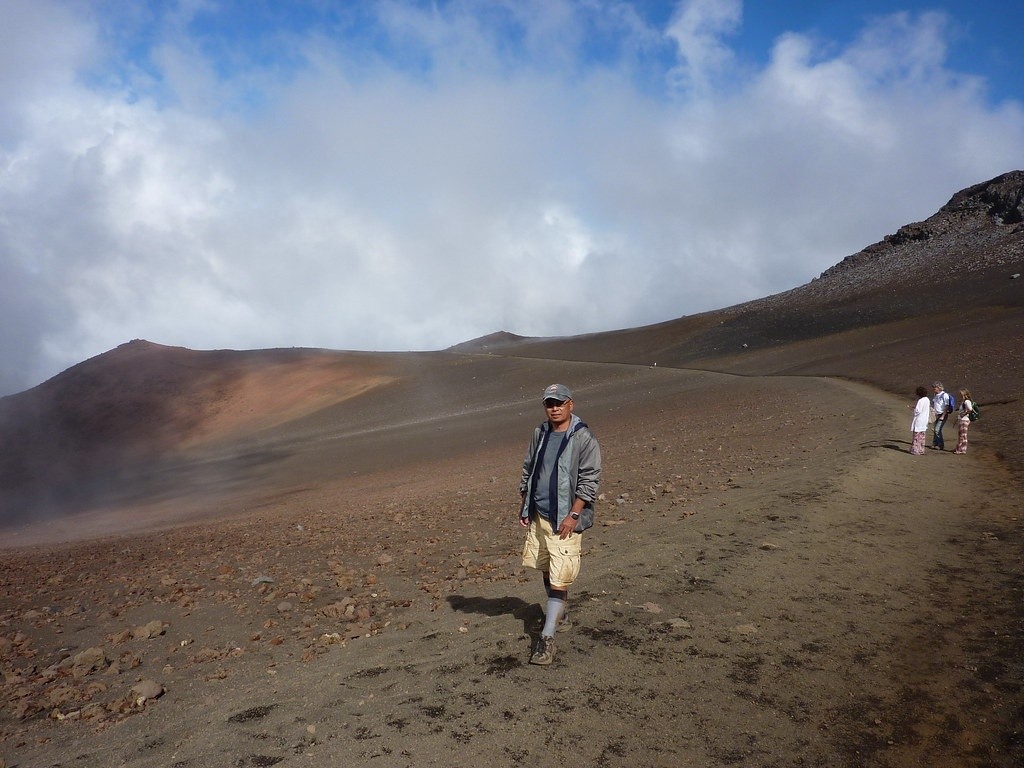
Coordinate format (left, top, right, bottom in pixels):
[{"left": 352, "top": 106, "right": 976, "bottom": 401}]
[{"left": 543, "top": 399, "right": 570, "bottom": 408}]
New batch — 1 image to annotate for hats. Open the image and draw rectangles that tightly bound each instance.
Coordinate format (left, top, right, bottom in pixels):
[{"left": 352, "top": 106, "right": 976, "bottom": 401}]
[{"left": 542, "top": 383, "right": 573, "bottom": 404}]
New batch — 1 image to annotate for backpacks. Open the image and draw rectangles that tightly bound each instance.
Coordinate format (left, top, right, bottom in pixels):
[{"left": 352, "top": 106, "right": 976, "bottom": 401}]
[
  {"left": 939, "top": 393, "right": 956, "bottom": 414},
  {"left": 963, "top": 399, "right": 981, "bottom": 421}
]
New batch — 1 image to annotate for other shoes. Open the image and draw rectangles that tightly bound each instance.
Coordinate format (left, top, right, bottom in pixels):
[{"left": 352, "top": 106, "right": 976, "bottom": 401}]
[
  {"left": 929, "top": 444, "right": 937, "bottom": 449},
  {"left": 953, "top": 450, "right": 967, "bottom": 455},
  {"left": 935, "top": 445, "right": 943, "bottom": 450}
]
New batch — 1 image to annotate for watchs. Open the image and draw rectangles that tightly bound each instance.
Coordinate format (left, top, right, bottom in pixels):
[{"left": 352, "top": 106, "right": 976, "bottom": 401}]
[{"left": 569, "top": 510, "right": 580, "bottom": 521}]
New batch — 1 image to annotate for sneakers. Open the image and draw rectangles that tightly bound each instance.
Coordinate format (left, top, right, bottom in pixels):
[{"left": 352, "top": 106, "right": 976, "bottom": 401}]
[
  {"left": 555, "top": 602, "right": 573, "bottom": 633},
  {"left": 528, "top": 633, "right": 558, "bottom": 665}
]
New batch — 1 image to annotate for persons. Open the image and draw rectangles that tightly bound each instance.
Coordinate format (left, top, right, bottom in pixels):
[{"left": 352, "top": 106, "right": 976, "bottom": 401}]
[
  {"left": 907, "top": 387, "right": 930, "bottom": 455},
  {"left": 519, "top": 384, "right": 601, "bottom": 665},
  {"left": 931, "top": 381, "right": 949, "bottom": 450},
  {"left": 953, "top": 387, "right": 973, "bottom": 454}
]
